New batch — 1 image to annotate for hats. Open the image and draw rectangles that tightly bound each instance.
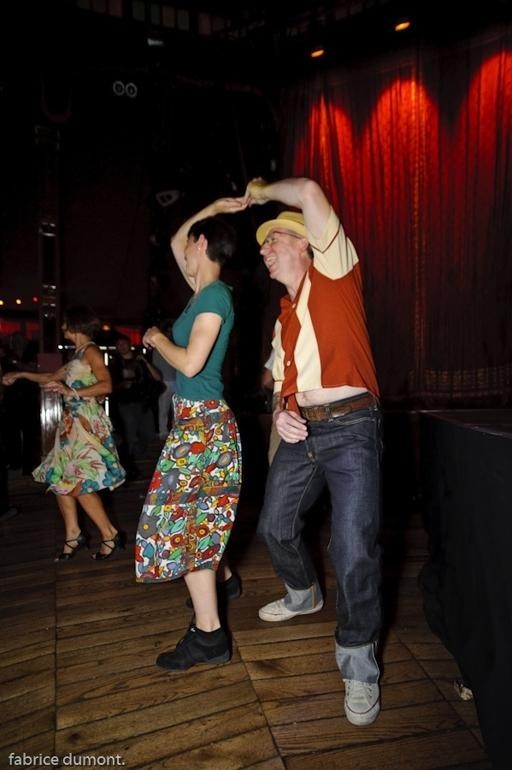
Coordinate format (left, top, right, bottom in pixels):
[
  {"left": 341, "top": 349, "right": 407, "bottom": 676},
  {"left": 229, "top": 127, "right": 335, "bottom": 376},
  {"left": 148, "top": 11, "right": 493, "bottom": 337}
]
[{"left": 255, "top": 211, "right": 308, "bottom": 246}]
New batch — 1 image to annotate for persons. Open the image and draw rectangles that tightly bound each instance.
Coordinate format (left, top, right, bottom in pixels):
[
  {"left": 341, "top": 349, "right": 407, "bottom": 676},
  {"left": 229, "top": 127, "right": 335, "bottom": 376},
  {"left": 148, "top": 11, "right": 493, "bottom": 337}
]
[
  {"left": 142, "top": 176, "right": 403, "bottom": 727},
  {"left": 3, "top": 300, "right": 127, "bottom": 560},
  {"left": 109, "top": 335, "right": 162, "bottom": 438}
]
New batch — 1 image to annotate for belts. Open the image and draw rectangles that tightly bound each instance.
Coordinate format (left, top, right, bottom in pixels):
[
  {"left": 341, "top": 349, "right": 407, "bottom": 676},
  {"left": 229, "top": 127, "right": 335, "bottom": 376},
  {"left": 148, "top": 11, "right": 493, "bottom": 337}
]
[{"left": 295, "top": 395, "right": 375, "bottom": 420}]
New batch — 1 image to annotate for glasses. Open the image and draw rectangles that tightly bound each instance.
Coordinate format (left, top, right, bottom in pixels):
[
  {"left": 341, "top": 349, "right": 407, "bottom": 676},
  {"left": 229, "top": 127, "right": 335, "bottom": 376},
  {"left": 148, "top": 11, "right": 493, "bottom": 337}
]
[{"left": 263, "top": 230, "right": 302, "bottom": 244}]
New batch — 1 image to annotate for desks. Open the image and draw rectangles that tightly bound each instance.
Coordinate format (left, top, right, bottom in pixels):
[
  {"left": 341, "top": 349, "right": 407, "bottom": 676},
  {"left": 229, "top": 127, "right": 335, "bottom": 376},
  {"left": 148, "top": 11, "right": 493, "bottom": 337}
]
[{"left": 416, "top": 409, "right": 512, "bottom": 770}]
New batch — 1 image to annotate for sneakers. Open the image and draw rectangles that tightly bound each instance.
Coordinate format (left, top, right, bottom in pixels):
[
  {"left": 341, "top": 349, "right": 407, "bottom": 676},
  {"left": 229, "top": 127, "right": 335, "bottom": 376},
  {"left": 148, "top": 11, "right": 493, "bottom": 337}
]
[
  {"left": 342, "top": 678, "right": 380, "bottom": 726},
  {"left": 186, "top": 573, "right": 241, "bottom": 608},
  {"left": 258, "top": 599, "right": 324, "bottom": 622},
  {"left": 156, "top": 625, "right": 229, "bottom": 669}
]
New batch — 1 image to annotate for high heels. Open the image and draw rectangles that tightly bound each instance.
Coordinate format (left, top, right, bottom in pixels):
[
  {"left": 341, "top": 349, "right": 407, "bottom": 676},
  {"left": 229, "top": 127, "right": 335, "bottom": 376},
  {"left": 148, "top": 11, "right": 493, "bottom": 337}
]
[{"left": 53, "top": 529, "right": 127, "bottom": 563}]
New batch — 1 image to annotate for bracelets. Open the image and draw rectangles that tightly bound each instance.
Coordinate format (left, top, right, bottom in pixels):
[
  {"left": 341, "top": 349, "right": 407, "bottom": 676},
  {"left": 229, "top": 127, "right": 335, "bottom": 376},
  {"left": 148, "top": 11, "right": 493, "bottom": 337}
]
[{"left": 71, "top": 387, "right": 81, "bottom": 401}]
